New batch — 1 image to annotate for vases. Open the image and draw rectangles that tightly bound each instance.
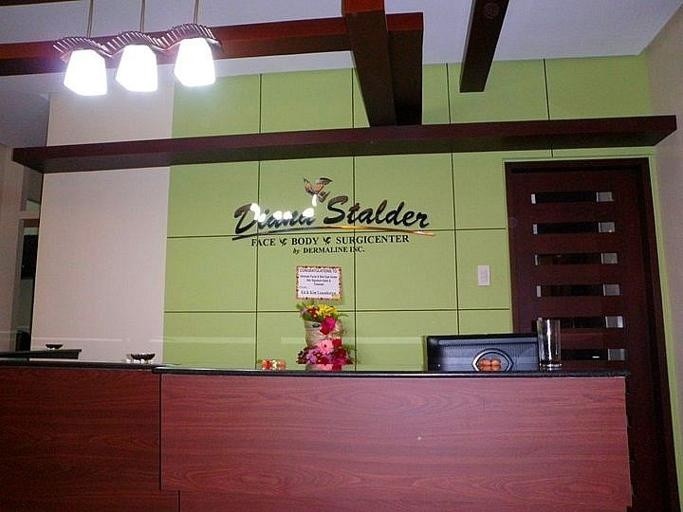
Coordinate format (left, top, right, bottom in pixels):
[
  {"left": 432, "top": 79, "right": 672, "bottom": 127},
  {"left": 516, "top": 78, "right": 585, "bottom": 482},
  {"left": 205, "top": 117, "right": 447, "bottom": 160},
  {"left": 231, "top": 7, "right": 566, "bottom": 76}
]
[{"left": 304, "top": 320, "right": 342, "bottom": 371}]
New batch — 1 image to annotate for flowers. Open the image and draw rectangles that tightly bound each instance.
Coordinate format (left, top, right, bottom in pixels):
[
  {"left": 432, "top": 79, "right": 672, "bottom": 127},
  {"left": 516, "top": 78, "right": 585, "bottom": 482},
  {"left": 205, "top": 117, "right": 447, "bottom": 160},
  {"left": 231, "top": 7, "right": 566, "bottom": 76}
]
[{"left": 296, "top": 293, "right": 362, "bottom": 373}]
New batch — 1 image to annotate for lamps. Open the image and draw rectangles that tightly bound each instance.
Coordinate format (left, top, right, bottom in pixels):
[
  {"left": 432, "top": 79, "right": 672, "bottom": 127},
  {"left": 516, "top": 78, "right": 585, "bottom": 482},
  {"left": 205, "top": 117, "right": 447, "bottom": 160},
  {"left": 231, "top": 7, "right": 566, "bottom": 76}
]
[{"left": 51, "top": 0, "right": 222, "bottom": 97}]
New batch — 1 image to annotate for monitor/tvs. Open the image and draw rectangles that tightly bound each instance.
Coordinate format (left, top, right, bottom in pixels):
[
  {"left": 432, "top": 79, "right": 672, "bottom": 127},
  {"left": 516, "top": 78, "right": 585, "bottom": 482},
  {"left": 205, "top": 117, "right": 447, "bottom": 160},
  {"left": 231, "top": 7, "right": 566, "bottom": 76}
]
[{"left": 426, "top": 332, "right": 544, "bottom": 373}]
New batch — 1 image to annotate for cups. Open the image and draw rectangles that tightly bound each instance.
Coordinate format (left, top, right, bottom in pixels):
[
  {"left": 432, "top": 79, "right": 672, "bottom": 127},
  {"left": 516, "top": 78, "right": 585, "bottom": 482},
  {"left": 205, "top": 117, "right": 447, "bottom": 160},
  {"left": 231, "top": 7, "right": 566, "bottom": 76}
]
[{"left": 535, "top": 316, "right": 564, "bottom": 369}]
